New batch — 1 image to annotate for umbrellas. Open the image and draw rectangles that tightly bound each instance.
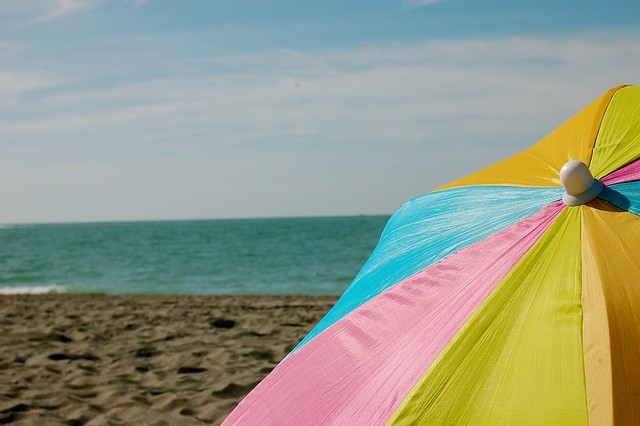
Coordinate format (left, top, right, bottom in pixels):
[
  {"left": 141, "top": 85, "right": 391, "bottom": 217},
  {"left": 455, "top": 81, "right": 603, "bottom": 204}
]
[{"left": 220, "top": 84, "right": 639, "bottom": 425}]
